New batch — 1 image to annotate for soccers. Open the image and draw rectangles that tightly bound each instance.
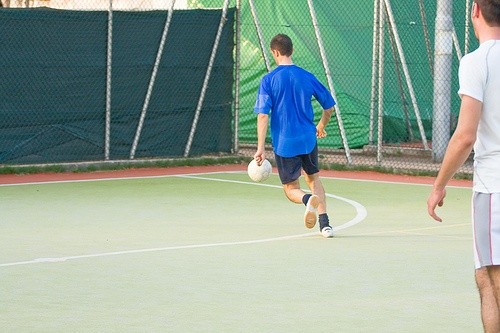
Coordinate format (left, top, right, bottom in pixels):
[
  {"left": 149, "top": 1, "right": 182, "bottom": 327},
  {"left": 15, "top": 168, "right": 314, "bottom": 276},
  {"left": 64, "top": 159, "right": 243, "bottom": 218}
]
[{"left": 247, "top": 157, "right": 272, "bottom": 182}]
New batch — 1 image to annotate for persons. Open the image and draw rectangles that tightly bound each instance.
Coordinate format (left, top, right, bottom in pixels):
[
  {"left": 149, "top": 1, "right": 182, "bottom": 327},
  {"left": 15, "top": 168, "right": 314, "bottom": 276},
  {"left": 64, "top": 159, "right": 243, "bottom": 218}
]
[
  {"left": 253, "top": 34, "right": 336, "bottom": 237},
  {"left": 427, "top": 0, "right": 500, "bottom": 333}
]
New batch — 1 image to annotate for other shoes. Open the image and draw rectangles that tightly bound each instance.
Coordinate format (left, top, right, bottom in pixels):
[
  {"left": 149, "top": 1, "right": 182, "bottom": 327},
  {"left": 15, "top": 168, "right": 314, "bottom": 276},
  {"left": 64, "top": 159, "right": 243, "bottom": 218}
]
[
  {"left": 319, "top": 227, "right": 334, "bottom": 237},
  {"left": 304, "top": 194, "right": 320, "bottom": 228}
]
